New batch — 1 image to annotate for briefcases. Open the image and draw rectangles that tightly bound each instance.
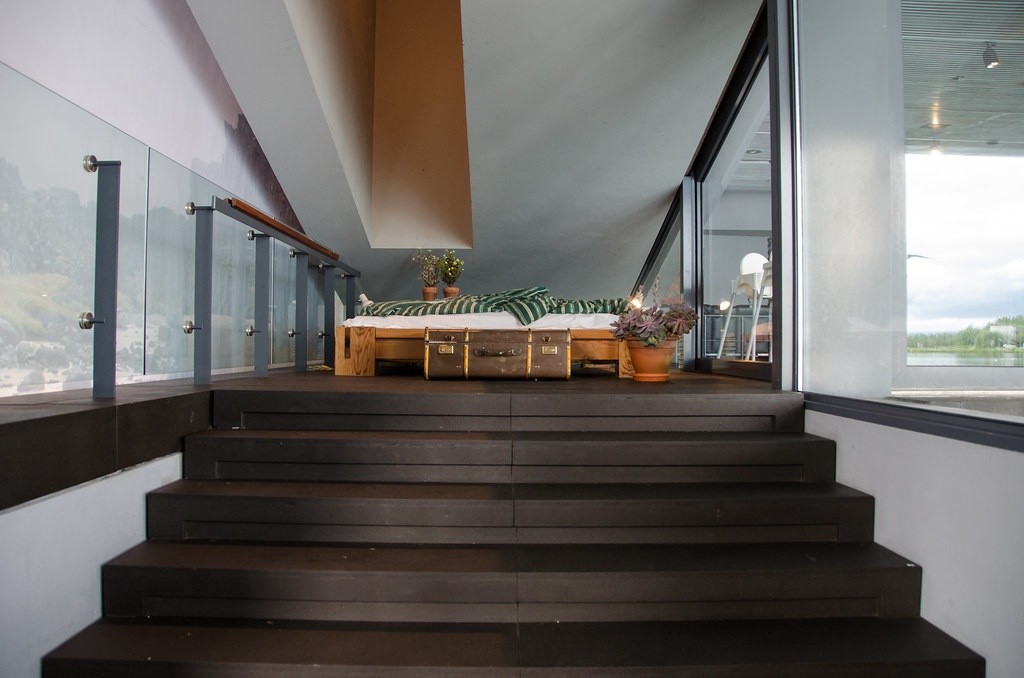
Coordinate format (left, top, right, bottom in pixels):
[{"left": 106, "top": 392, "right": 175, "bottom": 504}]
[{"left": 423, "top": 325, "right": 571, "bottom": 381}]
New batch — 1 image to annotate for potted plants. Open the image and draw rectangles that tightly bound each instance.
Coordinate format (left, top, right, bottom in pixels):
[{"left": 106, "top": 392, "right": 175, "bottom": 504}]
[{"left": 609, "top": 276, "right": 699, "bottom": 382}]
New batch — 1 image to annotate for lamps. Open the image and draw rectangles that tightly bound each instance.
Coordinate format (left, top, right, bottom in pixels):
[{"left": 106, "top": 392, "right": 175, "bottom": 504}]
[{"left": 983, "top": 41, "right": 999, "bottom": 70}]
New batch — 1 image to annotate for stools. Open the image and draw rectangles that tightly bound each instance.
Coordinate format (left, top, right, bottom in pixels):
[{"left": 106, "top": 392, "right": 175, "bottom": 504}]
[{"left": 715, "top": 252, "right": 773, "bottom": 362}]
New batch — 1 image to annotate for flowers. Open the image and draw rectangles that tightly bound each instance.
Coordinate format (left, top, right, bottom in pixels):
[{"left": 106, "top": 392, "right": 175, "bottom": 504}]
[
  {"left": 437, "top": 249, "right": 464, "bottom": 286},
  {"left": 412, "top": 247, "right": 441, "bottom": 287}
]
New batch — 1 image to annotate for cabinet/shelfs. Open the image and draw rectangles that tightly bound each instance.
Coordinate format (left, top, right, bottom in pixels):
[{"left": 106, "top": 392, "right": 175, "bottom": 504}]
[{"left": 703, "top": 314, "right": 769, "bottom": 358}]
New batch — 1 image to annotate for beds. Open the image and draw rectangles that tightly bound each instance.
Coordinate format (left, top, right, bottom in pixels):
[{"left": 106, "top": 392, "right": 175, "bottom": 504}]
[{"left": 334, "top": 286, "right": 635, "bottom": 378}]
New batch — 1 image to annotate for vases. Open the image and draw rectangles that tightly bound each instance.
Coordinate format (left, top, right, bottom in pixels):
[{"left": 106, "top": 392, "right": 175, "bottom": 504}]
[
  {"left": 443, "top": 287, "right": 460, "bottom": 297},
  {"left": 422, "top": 287, "right": 439, "bottom": 300}
]
